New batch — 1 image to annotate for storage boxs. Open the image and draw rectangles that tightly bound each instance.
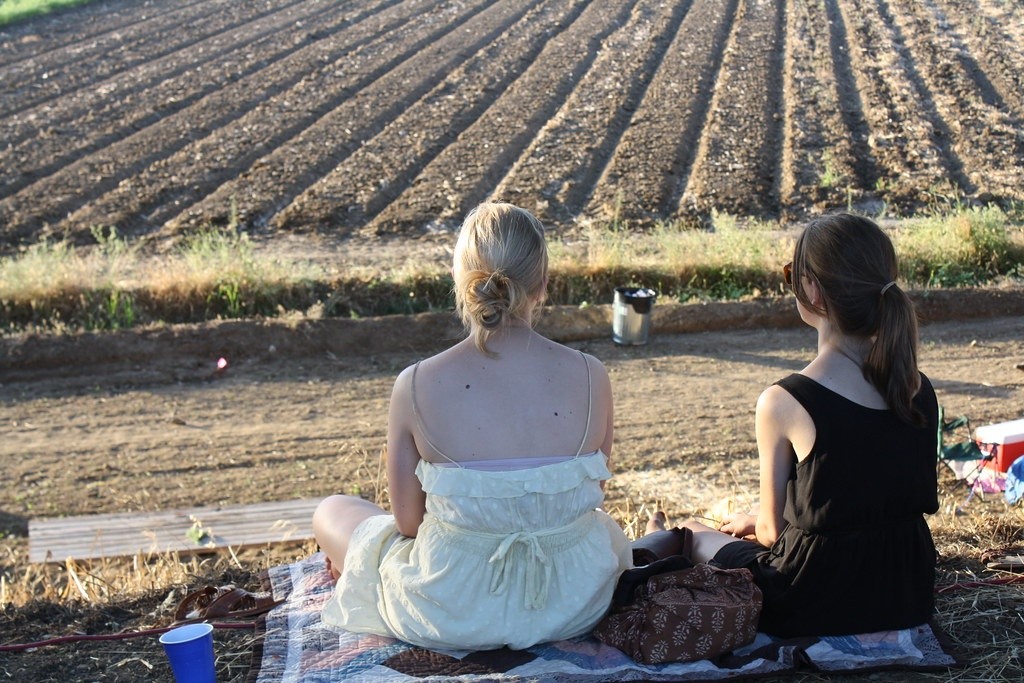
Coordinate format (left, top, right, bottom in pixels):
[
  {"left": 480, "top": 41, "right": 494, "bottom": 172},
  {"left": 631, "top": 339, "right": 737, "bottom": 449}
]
[{"left": 974, "top": 419, "right": 1024, "bottom": 474}]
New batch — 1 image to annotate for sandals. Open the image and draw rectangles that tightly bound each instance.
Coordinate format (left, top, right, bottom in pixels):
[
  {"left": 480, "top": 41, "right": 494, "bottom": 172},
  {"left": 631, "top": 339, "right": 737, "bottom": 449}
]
[{"left": 165, "top": 586, "right": 282, "bottom": 627}]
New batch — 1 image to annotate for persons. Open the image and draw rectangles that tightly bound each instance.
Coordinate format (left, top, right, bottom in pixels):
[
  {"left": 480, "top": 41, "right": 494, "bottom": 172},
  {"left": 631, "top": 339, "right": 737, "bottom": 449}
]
[
  {"left": 313, "top": 199, "right": 634, "bottom": 649},
  {"left": 642, "top": 213, "right": 941, "bottom": 642}
]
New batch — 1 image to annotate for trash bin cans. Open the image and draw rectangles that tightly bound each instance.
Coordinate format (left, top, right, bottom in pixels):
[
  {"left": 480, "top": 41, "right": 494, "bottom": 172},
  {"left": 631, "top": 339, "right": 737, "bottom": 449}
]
[{"left": 612, "top": 287, "right": 658, "bottom": 346}]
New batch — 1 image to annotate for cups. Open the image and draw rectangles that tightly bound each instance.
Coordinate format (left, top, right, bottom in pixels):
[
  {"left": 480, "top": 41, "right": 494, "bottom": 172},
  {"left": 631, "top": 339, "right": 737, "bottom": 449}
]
[{"left": 159, "top": 623, "right": 217, "bottom": 683}]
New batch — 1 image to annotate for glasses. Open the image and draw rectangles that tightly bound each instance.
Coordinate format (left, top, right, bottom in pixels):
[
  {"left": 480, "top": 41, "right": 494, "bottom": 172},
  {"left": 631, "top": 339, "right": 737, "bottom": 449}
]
[{"left": 782, "top": 261, "right": 813, "bottom": 281}]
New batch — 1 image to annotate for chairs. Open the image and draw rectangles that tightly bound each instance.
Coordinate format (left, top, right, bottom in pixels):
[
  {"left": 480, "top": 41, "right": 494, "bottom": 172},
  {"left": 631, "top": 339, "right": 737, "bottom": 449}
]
[{"left": 938, "top": 406, "right": 998, "bottom": 507}]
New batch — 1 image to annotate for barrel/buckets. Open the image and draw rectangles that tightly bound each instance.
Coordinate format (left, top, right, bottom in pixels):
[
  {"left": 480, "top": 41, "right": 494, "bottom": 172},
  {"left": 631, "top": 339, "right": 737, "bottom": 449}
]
[{"left": 612, "top": 287, "right": 655, "bottom": 345}]
